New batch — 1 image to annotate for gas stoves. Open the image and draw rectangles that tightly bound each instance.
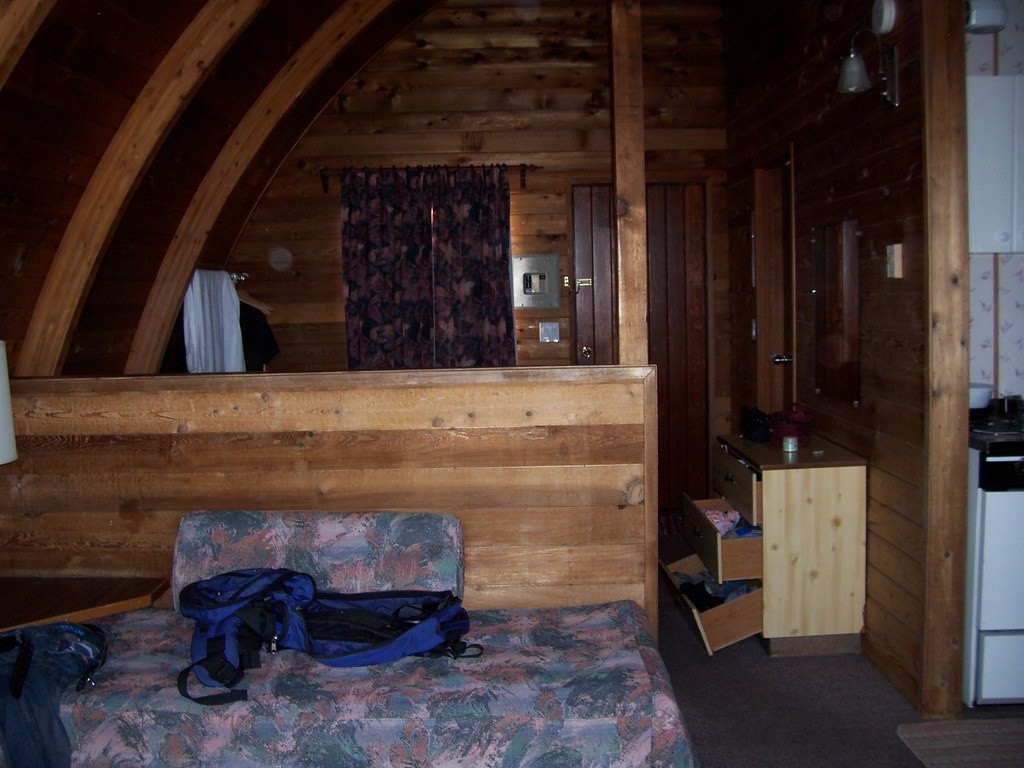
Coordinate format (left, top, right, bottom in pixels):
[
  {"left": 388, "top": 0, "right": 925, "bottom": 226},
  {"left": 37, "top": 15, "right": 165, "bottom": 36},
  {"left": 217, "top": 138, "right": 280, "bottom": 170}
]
[{"left": 969, "top": 397, "right": 1024, "bottom": 456}]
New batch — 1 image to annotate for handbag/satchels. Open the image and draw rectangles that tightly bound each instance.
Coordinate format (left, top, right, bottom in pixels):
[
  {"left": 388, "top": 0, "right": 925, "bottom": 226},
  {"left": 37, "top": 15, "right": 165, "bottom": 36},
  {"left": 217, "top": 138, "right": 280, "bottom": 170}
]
[
  {"left": 768, "top": 402, "right": 817, "bottom": 449},
  {"left": 738, "top": 405, "right": 770, "bottom": 442},
  {"left": 178, "top": 568, "right": 484, "bottom": 705}
]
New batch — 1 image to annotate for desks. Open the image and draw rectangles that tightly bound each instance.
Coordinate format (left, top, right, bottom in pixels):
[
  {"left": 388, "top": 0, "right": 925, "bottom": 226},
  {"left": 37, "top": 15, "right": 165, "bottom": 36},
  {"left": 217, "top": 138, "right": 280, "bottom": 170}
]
[{"left": 0, "top": 577, "right": 170, "bottom": 632}]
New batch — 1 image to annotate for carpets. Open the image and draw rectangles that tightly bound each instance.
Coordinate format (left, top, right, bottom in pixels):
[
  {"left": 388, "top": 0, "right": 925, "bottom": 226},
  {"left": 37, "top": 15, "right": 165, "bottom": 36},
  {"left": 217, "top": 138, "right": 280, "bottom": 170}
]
[{"left": 897, "top": 718, "right": 1024, "bottom": 768}]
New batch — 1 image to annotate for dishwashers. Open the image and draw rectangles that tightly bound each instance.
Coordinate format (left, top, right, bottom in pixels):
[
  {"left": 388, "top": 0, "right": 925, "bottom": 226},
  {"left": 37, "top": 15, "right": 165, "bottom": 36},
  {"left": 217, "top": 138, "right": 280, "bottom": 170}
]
[{"left": 976, "top": 446, "right": 1024, "bottom": 702}]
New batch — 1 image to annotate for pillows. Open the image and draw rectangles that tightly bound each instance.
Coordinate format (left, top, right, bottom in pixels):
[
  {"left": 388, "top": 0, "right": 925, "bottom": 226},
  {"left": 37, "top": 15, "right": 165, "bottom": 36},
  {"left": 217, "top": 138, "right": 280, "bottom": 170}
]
[{"left": 172, "top": 510, "right": 465, "bottom": 614}]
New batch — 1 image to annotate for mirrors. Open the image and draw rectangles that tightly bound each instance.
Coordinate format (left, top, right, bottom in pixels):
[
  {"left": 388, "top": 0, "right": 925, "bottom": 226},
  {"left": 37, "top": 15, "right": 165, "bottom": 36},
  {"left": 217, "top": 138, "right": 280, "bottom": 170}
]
[{"left": 809, "top": 218, "right": 863, "bottom": 409}]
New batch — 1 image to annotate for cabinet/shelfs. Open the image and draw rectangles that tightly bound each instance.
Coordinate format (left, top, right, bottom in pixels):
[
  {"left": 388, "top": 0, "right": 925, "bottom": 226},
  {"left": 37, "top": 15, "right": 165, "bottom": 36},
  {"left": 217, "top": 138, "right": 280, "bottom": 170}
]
[
  {"left": 658, "top": 431, "right": 867, "bottom": 657},
  {"left": 966, "top": 75, "right": 1024, "bottom": 253},
  {"left": 961, "top": 436, "right": 1024, "bottom": 708}
]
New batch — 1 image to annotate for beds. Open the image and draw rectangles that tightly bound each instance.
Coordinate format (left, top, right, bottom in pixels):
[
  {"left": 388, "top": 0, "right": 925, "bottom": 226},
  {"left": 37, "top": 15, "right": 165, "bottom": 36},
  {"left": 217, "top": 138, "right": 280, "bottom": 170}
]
[{"left": 0, "top": 510, "right": 698, "bottom": 768}]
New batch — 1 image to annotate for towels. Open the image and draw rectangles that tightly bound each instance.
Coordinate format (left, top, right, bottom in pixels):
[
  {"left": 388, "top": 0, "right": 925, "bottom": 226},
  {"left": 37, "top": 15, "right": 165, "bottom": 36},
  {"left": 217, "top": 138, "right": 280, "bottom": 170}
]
[{"left": 184, "top": 270, "right": 246, "bottom": 373}]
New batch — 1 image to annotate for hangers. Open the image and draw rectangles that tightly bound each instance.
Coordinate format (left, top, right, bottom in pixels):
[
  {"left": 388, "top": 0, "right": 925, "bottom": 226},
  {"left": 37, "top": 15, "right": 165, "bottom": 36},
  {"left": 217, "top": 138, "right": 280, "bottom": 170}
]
[{"left": 228, "top": 274, "right": 273, "bottom": 314}]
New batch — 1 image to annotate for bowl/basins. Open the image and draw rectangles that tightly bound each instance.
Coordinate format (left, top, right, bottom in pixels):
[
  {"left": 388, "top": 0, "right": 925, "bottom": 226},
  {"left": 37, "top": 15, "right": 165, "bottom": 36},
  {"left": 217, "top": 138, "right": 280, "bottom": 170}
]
[{"left": 969, "top": 383, "right": 996, "bottom": 410}]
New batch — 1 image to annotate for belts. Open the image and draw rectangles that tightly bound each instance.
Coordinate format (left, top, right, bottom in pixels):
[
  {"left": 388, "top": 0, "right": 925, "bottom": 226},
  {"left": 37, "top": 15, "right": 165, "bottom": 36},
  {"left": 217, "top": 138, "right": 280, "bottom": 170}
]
[{"left": 0, "top": 624, "right": 108, "bottom": 700}]
